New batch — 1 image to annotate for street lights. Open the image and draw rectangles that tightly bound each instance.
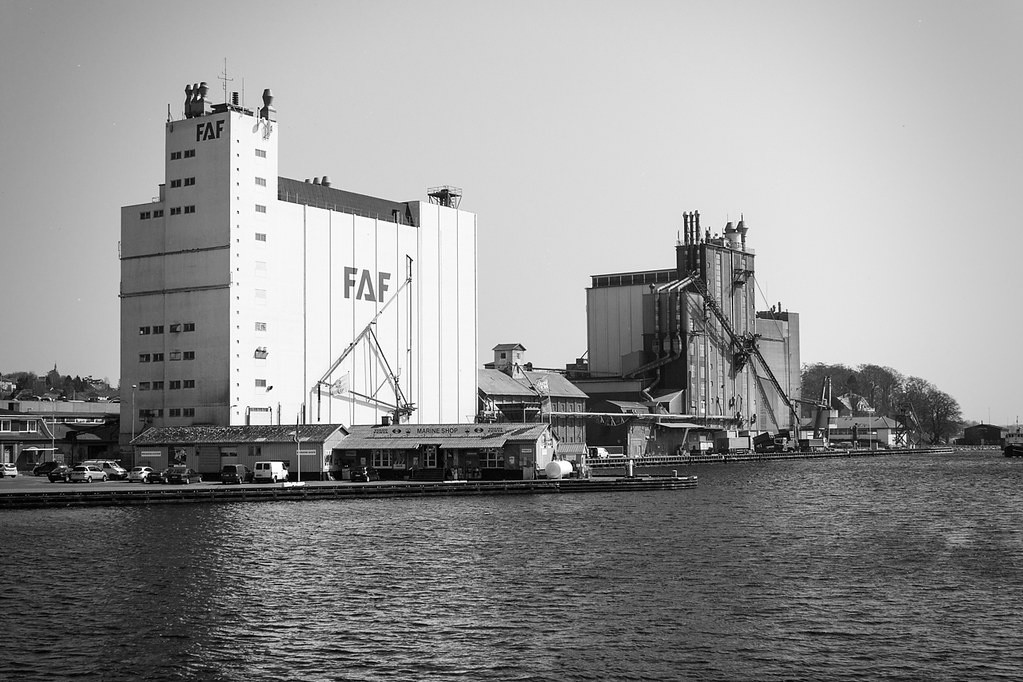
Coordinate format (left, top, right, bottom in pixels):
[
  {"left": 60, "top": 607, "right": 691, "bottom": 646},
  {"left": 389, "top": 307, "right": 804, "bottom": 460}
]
[{"left": 132, "top": 385, "right": 136, "bottom": 439}]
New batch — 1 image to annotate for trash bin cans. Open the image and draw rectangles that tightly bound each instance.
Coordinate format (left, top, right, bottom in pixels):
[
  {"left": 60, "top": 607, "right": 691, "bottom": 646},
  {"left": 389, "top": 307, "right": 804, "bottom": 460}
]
[
  {"left": 624, "top": 460, "right": 633, "bottom": 477},
  {"left": 871, "top": 443, "right": 878, "bottom": 450}
]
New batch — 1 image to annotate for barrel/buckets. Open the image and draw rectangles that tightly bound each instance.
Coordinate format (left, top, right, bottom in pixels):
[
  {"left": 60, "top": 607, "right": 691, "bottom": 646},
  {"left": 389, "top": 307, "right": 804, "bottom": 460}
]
[{"left": 545, "top": 461, "right": 573, "bottom": 478}]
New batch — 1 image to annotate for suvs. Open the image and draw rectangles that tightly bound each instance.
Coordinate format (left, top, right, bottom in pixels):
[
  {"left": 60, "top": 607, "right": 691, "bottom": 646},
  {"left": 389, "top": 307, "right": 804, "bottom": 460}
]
[
  {"left": 33, "top": 460, "right": 67, "bottom": 476},
  {"left": 0, "top": 463, "right": 18, "bottom": 478}
]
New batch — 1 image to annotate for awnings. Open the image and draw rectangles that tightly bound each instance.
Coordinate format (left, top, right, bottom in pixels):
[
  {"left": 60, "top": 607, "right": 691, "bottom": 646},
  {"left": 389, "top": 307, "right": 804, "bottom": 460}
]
[
  {"left": 333, "top": 439, "right": 420, "bottom": 449},
  {"left": 439, "top": 438, "right": 507, "bottom": 448}
]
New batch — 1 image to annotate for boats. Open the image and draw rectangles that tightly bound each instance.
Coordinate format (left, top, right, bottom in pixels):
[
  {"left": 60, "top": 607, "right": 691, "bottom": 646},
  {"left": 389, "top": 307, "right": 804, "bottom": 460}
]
[{"left": 1004, "top": 416, "right": 1023, "bottom": 457}]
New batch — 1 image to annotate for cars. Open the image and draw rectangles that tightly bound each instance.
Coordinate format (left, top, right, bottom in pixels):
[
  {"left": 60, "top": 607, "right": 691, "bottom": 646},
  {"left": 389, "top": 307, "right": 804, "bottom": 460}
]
[
  {"left": 48, "top": 465, "right": 108, "bottom": 483},
  {"left": 128, "top": 461, "right": 196, "bottom": 485},
  {"left": 350, "top": 466, "right": 380, "bottom": 482}
]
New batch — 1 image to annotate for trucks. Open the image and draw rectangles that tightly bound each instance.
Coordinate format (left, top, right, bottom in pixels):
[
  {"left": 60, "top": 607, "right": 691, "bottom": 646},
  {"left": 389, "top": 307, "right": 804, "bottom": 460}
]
[{"left": 221, "top": 465, "right": 253, "bottom": 484}]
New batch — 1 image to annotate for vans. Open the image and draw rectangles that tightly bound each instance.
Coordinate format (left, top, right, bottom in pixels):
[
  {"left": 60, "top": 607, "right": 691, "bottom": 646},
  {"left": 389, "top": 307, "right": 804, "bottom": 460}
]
[
  {"left": 587, "top": 447, "right": 609, "bottom": 459},
  {"left": 82, "top": 459, "right": 128, "bottom": 481},
  {"left": 254, "top": 461, "right": 289, "bottom": 483}
]
